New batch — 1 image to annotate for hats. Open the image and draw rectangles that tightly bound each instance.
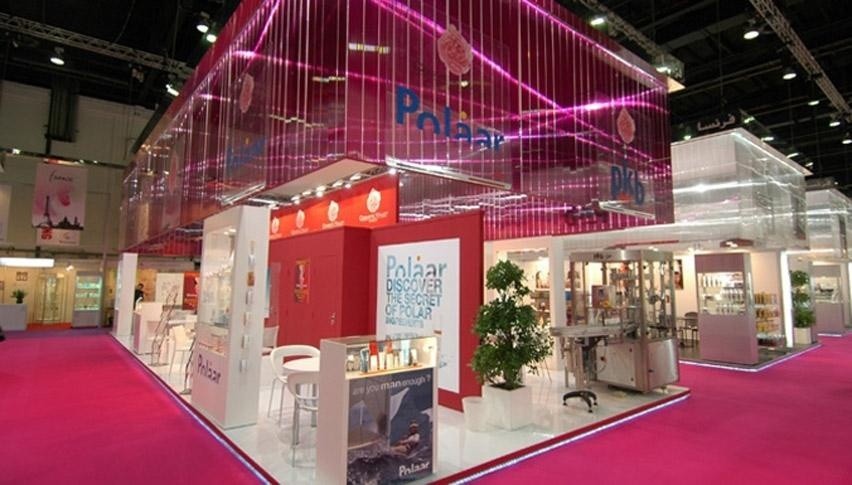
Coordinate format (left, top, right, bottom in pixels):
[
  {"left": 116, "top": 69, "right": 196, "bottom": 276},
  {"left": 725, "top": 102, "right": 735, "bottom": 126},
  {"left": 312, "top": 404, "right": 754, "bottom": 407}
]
[{"left": 408, "top": 420, "right": 419, "bottom": 429}]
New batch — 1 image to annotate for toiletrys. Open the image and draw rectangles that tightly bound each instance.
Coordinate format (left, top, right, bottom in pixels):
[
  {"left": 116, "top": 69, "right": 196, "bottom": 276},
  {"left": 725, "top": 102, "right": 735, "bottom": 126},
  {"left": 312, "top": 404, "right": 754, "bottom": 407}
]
[{"left": 361, "top": 340, "right": 418, "bottom": 373}]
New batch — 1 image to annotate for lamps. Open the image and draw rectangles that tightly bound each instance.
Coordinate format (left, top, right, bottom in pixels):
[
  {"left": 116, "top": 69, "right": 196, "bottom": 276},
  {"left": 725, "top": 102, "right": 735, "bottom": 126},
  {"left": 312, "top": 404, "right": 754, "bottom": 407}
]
[
  {"left": 49, "top": 44, "right": 65, "bottom": 64},
  {"left": 196, "top": 8, "right": 220, "bottom": 42},
  {"left": 165, "top": 72, "right": 184, "bottom": 96},
  {"left": 742, "top": 14, "right": 852, "bottom": 146}
]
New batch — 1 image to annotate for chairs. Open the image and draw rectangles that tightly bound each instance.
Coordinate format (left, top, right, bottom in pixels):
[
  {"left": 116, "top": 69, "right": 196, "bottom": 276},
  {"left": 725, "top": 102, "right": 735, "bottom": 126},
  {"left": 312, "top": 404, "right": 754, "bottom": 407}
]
[
  {"left": 263, "top": 327, "right": 279, "bottom": 354},
  {"left": 266, "top": 345, "right": 320, "bottom": 426},
  {"left": 685, "top": 310, "right": 698, "bottom": 347},
  {"left": 285, "top": 371, "right": 320, "bottom": 467},
  {"left": 169, "top": 325, "right": 195, "bottom": 375}
]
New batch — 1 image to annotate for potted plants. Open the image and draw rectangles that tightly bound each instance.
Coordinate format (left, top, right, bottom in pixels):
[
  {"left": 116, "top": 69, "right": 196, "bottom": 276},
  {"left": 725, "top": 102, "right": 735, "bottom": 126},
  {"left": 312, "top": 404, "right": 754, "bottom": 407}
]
[
  {"left": 9, "top": 288, "right": 27, "bottom": 304},
  {"left": 792, "top": 268, "right": 814, "bottom": 344},
  {"left": 472, "top": 260, "right": 551, "bottom": 431}
]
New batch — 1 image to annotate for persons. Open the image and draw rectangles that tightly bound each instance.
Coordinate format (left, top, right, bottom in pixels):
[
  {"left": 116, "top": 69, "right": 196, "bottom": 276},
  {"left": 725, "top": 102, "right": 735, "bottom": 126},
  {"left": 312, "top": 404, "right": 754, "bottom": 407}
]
[{"left": 136, "top": 283, "right": 144, "bottom": 302}]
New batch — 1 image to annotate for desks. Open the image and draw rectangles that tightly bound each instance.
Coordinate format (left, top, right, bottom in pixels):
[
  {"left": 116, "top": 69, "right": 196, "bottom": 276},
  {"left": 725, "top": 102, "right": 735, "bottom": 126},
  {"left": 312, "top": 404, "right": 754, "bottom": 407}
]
[
  {"left": 549, "top": 325, "right": 611, "bottom": 413},
  {"left": 281, "top": 360, "right": 319, "bottom": 444}
]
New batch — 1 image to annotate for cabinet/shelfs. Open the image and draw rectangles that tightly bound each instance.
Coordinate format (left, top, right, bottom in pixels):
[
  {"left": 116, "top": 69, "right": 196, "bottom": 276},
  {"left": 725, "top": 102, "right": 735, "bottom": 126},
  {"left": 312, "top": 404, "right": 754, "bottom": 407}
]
[
  {"left": 70, "top": 271, "right": 105, "bottom": 327},
  {"left": 695, "top": 252, "right": 758, "bottom": 364}
]
